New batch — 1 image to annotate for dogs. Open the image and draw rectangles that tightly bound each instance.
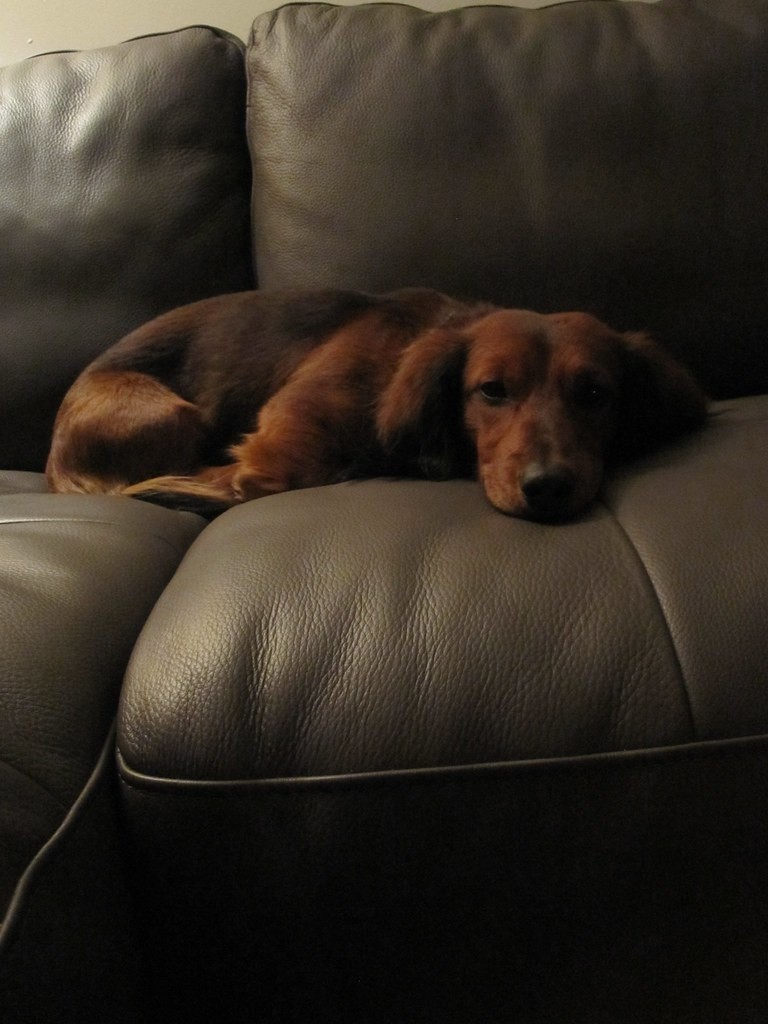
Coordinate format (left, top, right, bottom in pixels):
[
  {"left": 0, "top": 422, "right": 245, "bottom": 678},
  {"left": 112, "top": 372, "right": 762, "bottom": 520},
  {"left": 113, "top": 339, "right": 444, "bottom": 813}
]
[{"left": 48, "top": 288, "right": 715, "bottom": 524}]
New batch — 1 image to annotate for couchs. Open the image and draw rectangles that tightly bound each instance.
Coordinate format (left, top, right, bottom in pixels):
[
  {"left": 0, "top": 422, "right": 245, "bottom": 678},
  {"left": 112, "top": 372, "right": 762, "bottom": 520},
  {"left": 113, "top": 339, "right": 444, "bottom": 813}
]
[
  {"left": 113, "top": 0, "right": 768, "bottom": 1024},
  {"left": 0, "top": 23, "right": 253, "bottom": 1024}
]
